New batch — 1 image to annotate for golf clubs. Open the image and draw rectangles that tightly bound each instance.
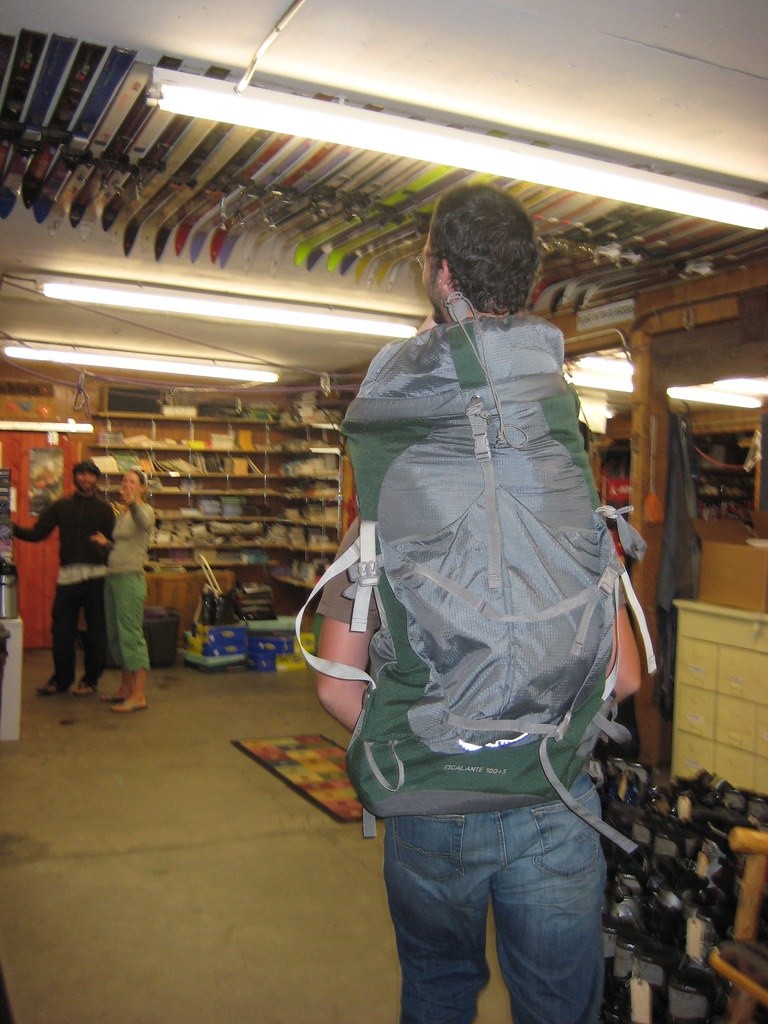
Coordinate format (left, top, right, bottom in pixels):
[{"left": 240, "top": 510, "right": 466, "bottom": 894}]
[{"left": 603, "top": 755, "right": 768, "bottom": 1024}]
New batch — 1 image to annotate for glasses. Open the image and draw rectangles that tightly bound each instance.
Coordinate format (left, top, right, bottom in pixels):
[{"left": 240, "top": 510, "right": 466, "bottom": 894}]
[{"left": 416, "top": 252, "right": 453, "bottom": 273}]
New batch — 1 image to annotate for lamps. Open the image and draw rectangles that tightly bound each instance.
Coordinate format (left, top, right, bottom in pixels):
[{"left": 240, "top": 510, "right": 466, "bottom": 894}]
[
  {"left": 0, "top": 339, "right": 281, "bottom": 383},
  {"left": 33, "top": 275, "right": 422, "bottom": 340},
  {"left": 145, "top": 64, "right": 768, "bottom": 232}
]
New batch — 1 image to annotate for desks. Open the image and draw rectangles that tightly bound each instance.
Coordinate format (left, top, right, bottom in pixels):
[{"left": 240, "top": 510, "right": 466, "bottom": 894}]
[
  {"left": 664, "top": 599, "right": 767, "bottom": 799},
  {"left": 140, "top": 568, "right": 232, "bottom": 638}
]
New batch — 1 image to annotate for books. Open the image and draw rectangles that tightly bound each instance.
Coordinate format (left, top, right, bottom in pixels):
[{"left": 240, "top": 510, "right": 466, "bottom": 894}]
[{"left": 92, "top": 390, "right": 340, "bottom": 584}]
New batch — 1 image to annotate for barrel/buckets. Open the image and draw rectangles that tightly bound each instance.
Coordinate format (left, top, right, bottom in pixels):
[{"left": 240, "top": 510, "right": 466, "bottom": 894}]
[{"left": 143, "top": 605, "right": 180, "bottom": 668}]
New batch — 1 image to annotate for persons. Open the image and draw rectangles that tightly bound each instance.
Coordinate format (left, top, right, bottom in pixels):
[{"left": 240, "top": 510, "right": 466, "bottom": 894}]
[
  {"left": 3, "top": 460, "right": 155, "bottom": 713},
  {"left": 314, "top": 182, "right": 641, "bottom": 1024}
]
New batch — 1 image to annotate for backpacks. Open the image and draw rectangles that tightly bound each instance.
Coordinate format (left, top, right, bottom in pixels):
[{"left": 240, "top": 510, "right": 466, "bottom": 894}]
[{"left": 336, "top": 312, "right": 624, "bottom": 816}]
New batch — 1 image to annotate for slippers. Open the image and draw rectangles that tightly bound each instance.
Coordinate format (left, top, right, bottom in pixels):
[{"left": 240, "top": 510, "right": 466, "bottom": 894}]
[
  {"left": 113, "top": 701, "right": 148, "bottom": 714},
  {"left": 103, "top": 693, "right": 126, "bottom": 704}
]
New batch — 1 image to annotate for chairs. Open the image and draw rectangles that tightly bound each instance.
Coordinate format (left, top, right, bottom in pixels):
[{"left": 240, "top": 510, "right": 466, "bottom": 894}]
[{"left": 709, "top": 825, "right": 768, "bottom": 1024}]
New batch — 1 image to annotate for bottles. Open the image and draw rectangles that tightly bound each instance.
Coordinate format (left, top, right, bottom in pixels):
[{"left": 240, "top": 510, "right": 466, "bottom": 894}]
[
  {"left": 693, "top": 471, "right": 753, "bottom": 522},
  {"left": 0, "top": 563, "right": 19, "bottom": 618},
  {"left": 596, "top": 756, "right": 768, "bottom": 1024}
]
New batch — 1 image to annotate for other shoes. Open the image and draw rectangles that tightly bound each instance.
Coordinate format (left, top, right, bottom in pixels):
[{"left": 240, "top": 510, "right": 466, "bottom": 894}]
[
  {"left": 72, "top": 681, "right": 97, "bottom": 697},
  {"left": 37, "top": 680, "right": 67, "bottom": 696}
]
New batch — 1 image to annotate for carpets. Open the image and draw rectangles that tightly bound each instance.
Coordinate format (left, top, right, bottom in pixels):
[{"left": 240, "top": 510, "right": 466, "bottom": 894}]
[{"left": 229, "top": 734, "right": 376, "bottom": 824}]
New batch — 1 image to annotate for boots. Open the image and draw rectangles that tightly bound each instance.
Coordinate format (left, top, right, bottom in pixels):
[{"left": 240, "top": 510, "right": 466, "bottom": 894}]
[
  {"left": 199, "top": 591, "right": 217, "bottom": 625},
  {"left": 213, "top": 596, "right": 241, "bottom": 625}
]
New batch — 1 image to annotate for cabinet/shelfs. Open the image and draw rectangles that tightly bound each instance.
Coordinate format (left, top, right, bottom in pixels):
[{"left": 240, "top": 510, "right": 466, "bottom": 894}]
[{"left": 87, "top": 412, "right": 341, "bottom": 592}]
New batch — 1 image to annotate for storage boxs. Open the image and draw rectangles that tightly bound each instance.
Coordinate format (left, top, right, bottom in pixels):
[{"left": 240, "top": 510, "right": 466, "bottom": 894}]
[
  {"left": 223, "top": 457, "right": 249, "bottom": 477},
  {"left": 142, "top": 605, "right": 316, "bottom": 672},
  {"left": 239, "top": 430, "right": 252, "bottom": 449},
  {"left": 687, "top": 511, "right": 768, "bottom": 615}
]
